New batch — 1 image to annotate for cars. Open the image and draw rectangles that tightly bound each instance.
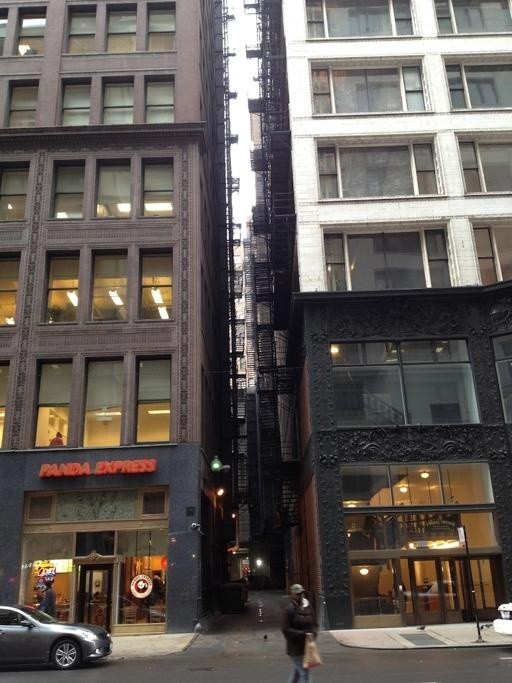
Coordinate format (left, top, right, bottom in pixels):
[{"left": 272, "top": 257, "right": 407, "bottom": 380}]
[{"left": 0, "top": 604, "right": 113, "bottom": 670}]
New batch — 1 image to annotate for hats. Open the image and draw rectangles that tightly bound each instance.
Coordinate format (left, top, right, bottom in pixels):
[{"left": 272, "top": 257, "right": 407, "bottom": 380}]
[{"left": 291, "top": 584, "right": 305, "bottom": 593}]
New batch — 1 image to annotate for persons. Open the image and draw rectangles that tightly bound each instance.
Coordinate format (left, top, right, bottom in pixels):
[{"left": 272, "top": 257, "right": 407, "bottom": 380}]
[
  {"left": 49, "top": 433, "right": 65, "bottom": 446},
  {"left": 281, "top": 584, "right": 319, "bottom": 683},
  {"left": 40, "top": 581, "right": 55, "bottom": 618}
]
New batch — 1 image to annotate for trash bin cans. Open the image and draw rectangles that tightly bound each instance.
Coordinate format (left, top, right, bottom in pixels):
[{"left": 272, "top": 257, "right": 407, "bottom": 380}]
[{"left": 217, "top": 577, "right": 250, "bottom": 615}]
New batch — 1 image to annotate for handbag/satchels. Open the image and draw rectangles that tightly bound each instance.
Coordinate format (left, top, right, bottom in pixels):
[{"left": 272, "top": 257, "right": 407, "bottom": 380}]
[{"left": 303, "top": 635, "right": 323, "bottom": 668}]
